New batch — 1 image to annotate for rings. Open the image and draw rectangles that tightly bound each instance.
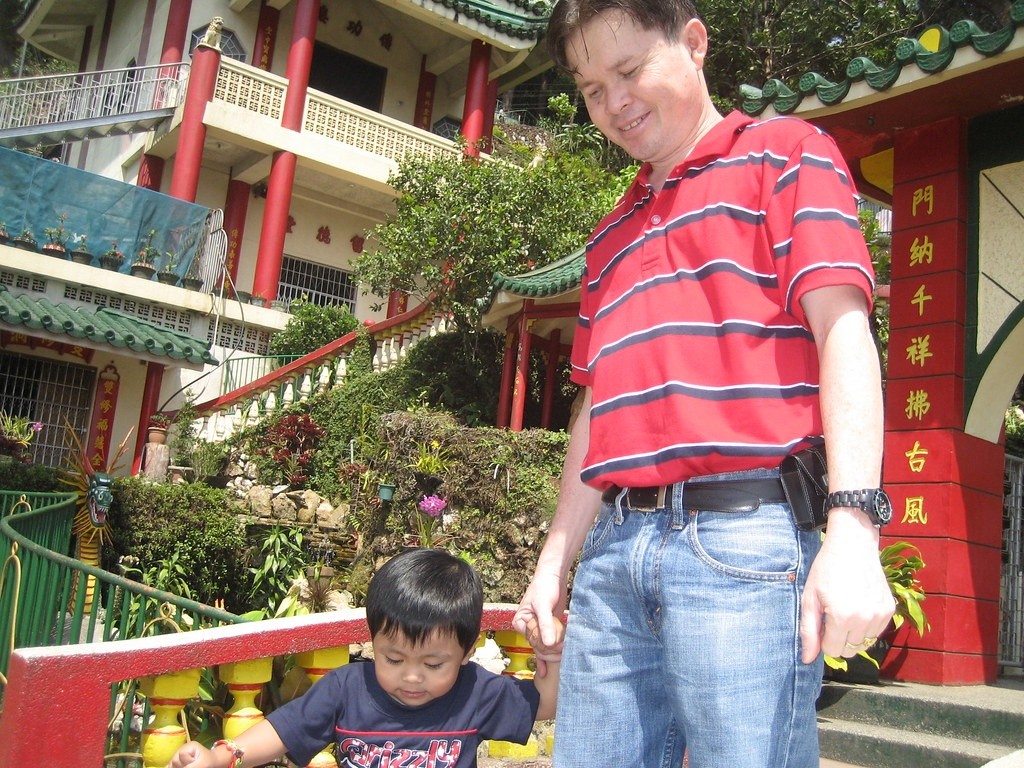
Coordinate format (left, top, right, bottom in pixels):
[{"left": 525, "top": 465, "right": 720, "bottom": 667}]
[
  {"left": 863, "top": 637, "right": 877, "bottom": 647},
  {"left": 845, "top": 641, "right": 863, "bottom": 650}
]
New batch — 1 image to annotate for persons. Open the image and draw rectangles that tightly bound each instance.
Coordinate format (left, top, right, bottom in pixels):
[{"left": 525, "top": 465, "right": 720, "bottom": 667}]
[
  {"left": 512, "top": 0, "right": 895, "bottom": 768},
  {"left": 168, "top": 549, "right": 565, "bottom": 768}
]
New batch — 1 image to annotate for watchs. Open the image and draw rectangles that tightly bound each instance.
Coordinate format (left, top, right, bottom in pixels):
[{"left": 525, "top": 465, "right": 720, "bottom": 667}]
[{"left": 823, "top": 488, "right": 893, "bottom": 529}]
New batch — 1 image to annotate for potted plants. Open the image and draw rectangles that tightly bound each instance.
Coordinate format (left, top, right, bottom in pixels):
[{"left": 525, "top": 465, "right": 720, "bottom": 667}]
[
  {"left": 250, "top": 291, "right": 267, "bottom": 307},
  {"left": 156, "top": 250, "right": 181, "bottom": 286},
  {"left": 289, "top": 293, "right": 308, "bottom": 315},
  {"left": 378, "top": 470, "right": 395, "bottom": 501},
  {"left": 11, "top": 223, "right": 38, "bottom": 251},
  {"left": 0, "top": 221, "right": 10, "bottom": 244},
  {"left": 824, "top": 541, "right": 932, "bottom": 691},
  {"left": 167, "top": 454, "right": 192, "bottom": 485}
]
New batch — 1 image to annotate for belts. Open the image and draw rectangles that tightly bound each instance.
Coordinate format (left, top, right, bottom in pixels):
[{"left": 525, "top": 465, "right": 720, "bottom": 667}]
[{"left": 600, "top": 479, "right": 790, "bottom": 514}]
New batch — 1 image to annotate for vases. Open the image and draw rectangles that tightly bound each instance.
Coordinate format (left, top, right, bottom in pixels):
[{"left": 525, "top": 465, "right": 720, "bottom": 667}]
[
  {"left": 148, "top": 427, "right": 168, "bottom": 444},
  {"left": 212, "top": 289, "right": 229, "bottom": 298},
  {"left": 306, "top": 566, "right": 334, "bottom": 590},
  {"left": 98, "top": 256, "right": 124, "bottom": 272},
  {"left": 270, "top": 300, "right": 289, "bottom": 313},
  {"left": 42, "top": 243, "right": 66, "bottom": 258},
  {"left": 233, "top": 291, "right": 251, "bottom": 304},
  {"left": 130, "top": 263, "right": 157, "bottom": 280},
  {"left": 69, "top": 250, "right": 94, "bottom": 265},
  {"left": 180, "top": 278, "right": 204, "bottom": 292}
]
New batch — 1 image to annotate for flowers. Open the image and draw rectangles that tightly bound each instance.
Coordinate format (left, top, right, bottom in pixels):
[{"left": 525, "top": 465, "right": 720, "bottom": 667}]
[
  {"left": 105, "top": 242, "right": 127, "bottom": 262},
  {"left": 0, "top": 408, "right": 44, "bottom": 449},
  {"left": 44, "top": 211, "right": 73, "bottom": 247},
  {"left": 148, "top": 412, "right": 171, "bottom": 429},
  {"left": 408, "top": 494, "right": 447, "bottom": 549},
  {"left": 78, "top": 235, "right": 88, "bottom": 252},
  {"left": 136, "top": 228, "right": 162, "bottom": 264}
]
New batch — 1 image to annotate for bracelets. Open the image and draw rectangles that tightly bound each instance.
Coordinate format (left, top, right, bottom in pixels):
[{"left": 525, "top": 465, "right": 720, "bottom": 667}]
[{"left": 211, "top": 740, "right": 244, "bottom": 768}]
